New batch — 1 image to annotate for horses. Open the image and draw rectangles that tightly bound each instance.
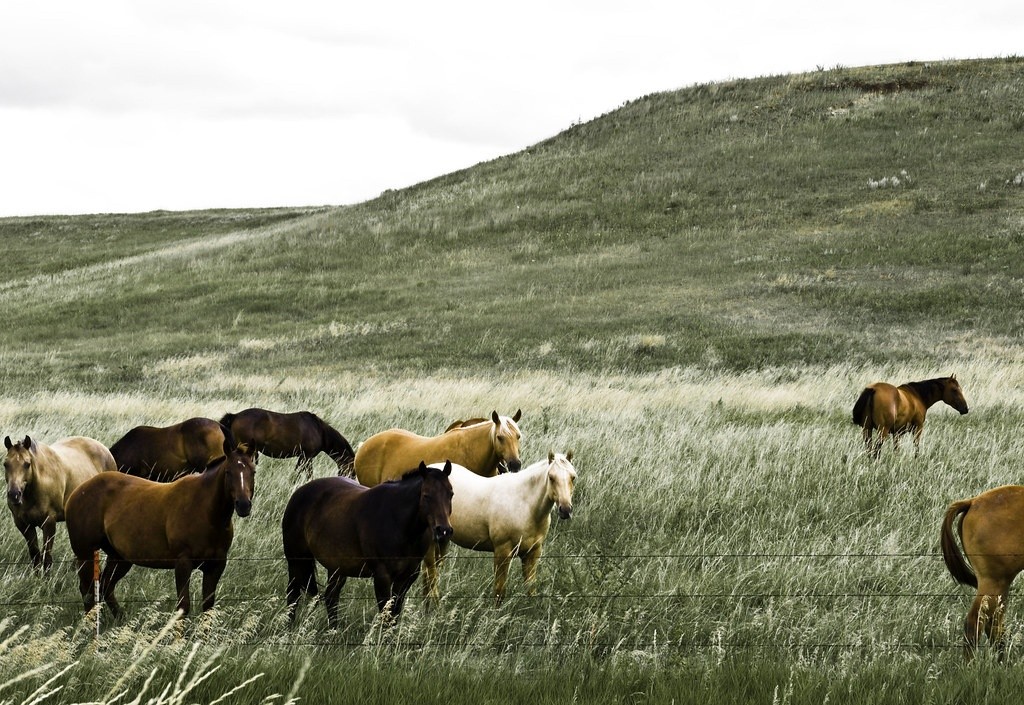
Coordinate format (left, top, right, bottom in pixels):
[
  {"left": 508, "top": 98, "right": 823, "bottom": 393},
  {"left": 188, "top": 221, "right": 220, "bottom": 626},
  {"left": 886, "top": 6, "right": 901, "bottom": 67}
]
[
  {"left": 220, "top": 407, "right": 356, "bottom": 480},
  {"left": 281, "top": 458, "right": 457, "bottom": 630},
  {"left": 63, "top": 439, "right": 258, "bottom": 617},
  {"left": 3, "top": 434, "right": 119, "bottom": 577},
  {"left": 852, "top": 373, "right": 972, "bottom": 460},
  {"left": 939, "top": 485, "right": 1024, "bottom": 660},
  {"left": 356, "top": 407, "right": 523, "bottom": 490},
  {"left": 424, "top": 448, "right": 579, "bottom": 598},
  {"left": 108, "top": 417, "right": 226, "bottom": 487}
]
